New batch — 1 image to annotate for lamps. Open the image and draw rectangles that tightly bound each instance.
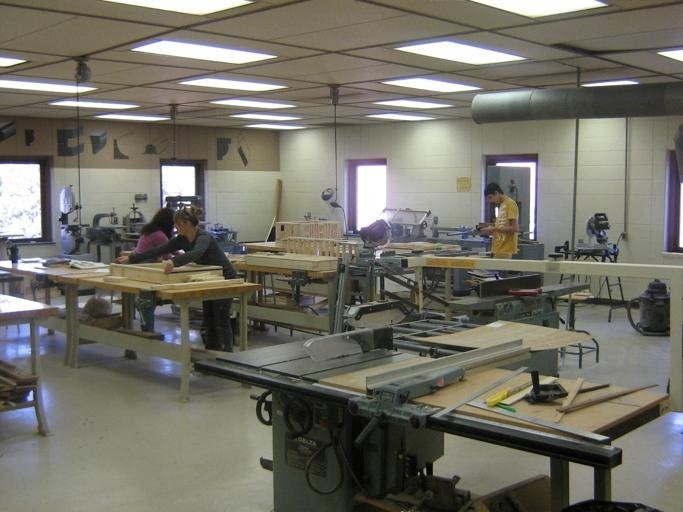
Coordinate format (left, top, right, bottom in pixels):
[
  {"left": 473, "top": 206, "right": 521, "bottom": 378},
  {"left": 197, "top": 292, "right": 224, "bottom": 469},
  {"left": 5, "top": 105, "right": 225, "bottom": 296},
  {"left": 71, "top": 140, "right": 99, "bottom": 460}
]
[{"left": 331, "top": 202, "right": 348, "bottom": 240}]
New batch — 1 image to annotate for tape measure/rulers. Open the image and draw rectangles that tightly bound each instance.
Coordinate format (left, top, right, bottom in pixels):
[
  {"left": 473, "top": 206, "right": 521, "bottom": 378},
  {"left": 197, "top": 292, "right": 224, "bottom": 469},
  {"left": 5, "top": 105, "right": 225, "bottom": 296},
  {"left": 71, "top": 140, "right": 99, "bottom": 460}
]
[{"left": 430, "top": 366, "right": 610, "bottom": 441}]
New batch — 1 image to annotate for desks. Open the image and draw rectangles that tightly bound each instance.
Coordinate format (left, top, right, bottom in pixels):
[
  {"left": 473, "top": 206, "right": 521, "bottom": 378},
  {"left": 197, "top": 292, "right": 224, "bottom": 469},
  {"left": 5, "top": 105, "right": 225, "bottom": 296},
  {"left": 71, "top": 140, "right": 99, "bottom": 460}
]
[{"left": 0, "top": 230, "right": 683, "bottom": 512}]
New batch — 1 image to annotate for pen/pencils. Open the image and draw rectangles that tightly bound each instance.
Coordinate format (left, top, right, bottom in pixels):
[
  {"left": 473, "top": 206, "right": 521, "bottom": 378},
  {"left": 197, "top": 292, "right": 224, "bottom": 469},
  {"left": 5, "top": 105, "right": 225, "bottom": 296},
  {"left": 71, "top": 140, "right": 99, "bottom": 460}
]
[{"left": 496, "top": 403, "right": 518, "bottom": 412}]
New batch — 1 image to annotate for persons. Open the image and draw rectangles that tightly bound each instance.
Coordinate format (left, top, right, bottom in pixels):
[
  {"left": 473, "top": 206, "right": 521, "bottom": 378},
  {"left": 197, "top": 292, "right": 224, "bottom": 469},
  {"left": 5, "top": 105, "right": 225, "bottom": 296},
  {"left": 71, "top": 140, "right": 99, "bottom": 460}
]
[
  {"left": 480, "top": 183, "right": 520, "bottom": 259},
  {"left": 113, "top": 209, "right": 236, "bottom": 353},
  {"left": 132, "top": 208, "right": 184, "bottom": 332}
]
